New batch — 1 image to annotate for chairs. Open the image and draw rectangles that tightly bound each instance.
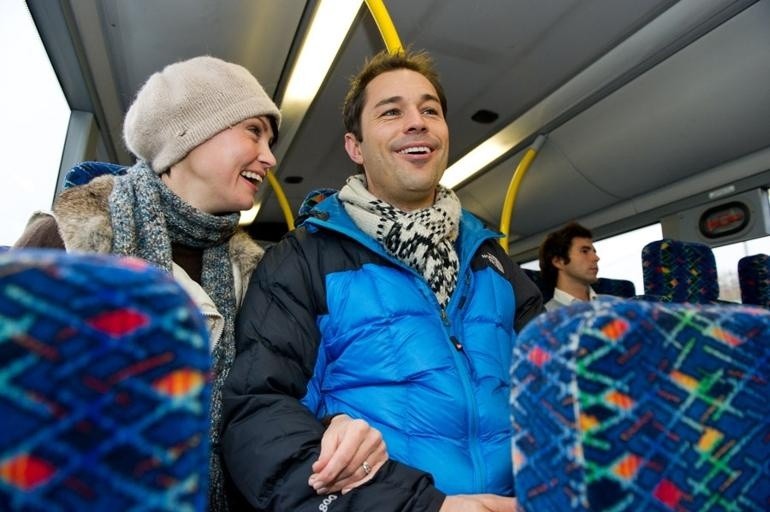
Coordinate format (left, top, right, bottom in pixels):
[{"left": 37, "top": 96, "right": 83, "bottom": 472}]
[
  {"left": 2, "top": 248, "right": 215, "bottom": 511},
  {"left": 520, "top": 268, "right": 637, "bottom": 303},
  {"left": 629, "top": 239, "right": 733, "bottom": 304},
  {"left": 738, "top": 253, "right": 770, "bottom": 309},
  {"left": 509, "top": 300, "right": 769, "bottom": 510}
]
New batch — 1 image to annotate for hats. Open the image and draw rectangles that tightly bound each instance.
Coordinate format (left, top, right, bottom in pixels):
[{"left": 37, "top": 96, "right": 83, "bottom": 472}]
[{"left": 123, "top": 56, "right": 280, "bottom": 175}]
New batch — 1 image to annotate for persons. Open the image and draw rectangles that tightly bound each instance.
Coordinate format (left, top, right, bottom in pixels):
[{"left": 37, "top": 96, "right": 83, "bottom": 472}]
[
  {"left": 539, "top": 222, "right": 622, "bottom": 311},
  {"left": 13, "top": 55, "right": 390, "bottom": 512},
  {"left": 218, "top": 49, "right": 547, "bottom": 512}
]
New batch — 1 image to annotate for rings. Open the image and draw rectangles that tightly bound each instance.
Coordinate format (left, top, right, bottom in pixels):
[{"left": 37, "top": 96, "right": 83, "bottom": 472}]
[{"left": 361, "top": 460, "right": 376, "bottom": 477}]
